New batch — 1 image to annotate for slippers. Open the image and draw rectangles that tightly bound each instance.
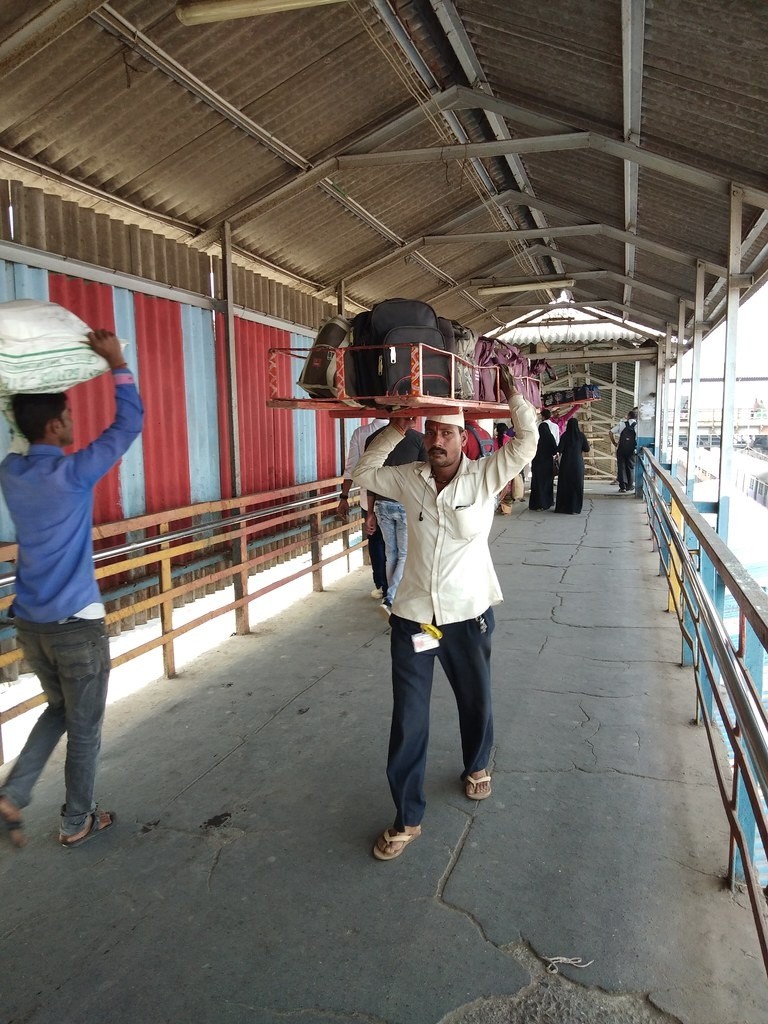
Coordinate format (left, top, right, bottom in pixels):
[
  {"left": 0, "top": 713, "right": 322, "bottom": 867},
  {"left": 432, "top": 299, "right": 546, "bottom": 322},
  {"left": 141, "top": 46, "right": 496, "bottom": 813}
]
[
  {"left": 466, "top": 768, "right": 491, "bottom": 800},
  {"left": 0, "top": 800, "right": 28, "bottom": 847},
  {"left": 374, "top": 825, "right": 421, "bottom": 860},
  {"left": 60, "top": 810, "right": 117, "bottom": 849}
]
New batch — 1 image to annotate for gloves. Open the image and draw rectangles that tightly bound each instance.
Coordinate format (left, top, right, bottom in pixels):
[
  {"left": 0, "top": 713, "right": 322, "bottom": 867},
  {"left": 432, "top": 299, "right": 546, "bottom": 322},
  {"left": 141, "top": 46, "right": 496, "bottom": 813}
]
[{"left": 495, "top": 362, "right": 516, "bottom": 391}]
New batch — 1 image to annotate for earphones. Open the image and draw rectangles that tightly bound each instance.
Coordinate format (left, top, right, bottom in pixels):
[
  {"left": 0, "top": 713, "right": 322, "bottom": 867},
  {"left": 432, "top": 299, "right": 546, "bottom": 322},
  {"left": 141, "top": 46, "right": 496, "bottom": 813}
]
[{"left": 419, "top": 512, "right": 423, "bottom": 521}]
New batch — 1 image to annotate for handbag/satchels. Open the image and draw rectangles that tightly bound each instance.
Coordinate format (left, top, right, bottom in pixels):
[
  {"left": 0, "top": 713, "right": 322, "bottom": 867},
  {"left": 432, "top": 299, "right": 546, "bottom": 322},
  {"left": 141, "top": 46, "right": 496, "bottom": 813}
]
[
  {"left": 541, "top": 384, "right": 599, "bottom": 407},
  {"left": 511, "top": 475, "right": 524, "bottom": 501},
  {"left": 552, "top": 459, "right": 560, "bottom": 476}
]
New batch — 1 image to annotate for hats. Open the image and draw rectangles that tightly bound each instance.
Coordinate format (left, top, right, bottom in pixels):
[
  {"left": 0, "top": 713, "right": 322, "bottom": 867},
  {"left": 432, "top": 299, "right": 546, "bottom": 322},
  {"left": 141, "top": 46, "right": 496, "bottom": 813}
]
[{"left": 425, "top": 410, "right": 465, "bottom": 431}]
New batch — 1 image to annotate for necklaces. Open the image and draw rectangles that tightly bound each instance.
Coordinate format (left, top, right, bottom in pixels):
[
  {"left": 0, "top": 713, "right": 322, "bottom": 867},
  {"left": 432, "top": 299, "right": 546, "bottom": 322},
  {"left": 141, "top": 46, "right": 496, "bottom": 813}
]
[{"left": 429, "top": 467, "right": 454, "bottom": 485}]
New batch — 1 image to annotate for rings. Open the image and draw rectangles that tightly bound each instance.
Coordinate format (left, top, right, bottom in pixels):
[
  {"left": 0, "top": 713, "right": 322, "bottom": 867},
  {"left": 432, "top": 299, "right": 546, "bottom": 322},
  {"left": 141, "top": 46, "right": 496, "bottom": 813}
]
[
  {"left": 102, "top": 331, "right": 108, "bottom": 335},
  {"left": 111, "top": 333, "right": 114, "bottom": 336},
  {"left": 90, "top": 335, "right": 96, "bottom": 341}
]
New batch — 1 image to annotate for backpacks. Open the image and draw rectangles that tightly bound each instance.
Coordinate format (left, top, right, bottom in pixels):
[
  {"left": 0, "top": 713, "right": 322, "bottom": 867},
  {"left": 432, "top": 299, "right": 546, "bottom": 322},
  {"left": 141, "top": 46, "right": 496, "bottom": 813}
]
[
  {"left": 617, "top": 421, "right": 637, "bottom": 457},
  {"left": 464, "top": 423, "right": 494, "bottom": 459},
  {"left": 295, "top": 293, "right": 559, "bottom": 414}
]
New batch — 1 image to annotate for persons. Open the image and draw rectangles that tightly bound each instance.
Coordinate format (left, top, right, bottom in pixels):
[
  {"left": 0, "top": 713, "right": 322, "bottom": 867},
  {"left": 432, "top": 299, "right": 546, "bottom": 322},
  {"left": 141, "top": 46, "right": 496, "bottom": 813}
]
[
  {"left": 461, "top": 418, "right": 525, "bottom": 515},
  {"left": 1, "top": 327, "right": 146, "bottom": 845},
  {"left": 529, "top": 402, "right": 590, "bottom": 516},
  {"left": 349, "top": 365, "right": 541, "bottom": 857},
  {"left": 337, "top": 416, "right": 429, "bottom": 619},
  {"left": 610, "top": 407, "right": 638, "bottom": 492}
]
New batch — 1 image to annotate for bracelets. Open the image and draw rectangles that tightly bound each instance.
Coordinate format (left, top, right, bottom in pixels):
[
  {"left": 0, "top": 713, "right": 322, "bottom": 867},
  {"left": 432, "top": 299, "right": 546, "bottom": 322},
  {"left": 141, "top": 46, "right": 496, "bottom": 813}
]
[
  {"left": 110, "top": 362, "right": 127, "bottom": 371},
  {"left": 339, "top": 494, "right": 349, "bottom": 499},
  {"left": 506, "top": 391, "right": 519, "bottom": 400},
  {"left": 391, "top": 421, "right": 406, "bottom": 438}
]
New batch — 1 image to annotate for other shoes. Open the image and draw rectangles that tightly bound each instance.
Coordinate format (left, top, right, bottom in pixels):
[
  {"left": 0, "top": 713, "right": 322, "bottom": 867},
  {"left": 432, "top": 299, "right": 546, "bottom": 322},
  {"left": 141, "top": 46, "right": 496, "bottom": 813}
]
[
  {"left": 610, "top": 480, "right": 621, "bottom": 485},
  {"left": 377, "top": 600, "right": 393, "bottom": 623},
  {"left": 619, "top": 489, "right": 626, "bottom": 493},
  {"left": 626, "top": 485, "right": 634, "bottom": 491},
  {"left": 370, "top": 588, "right": 383, "bottom": 600},
  {"left": 513, "top": 498, "right": 525, "bottom": 502}
]
